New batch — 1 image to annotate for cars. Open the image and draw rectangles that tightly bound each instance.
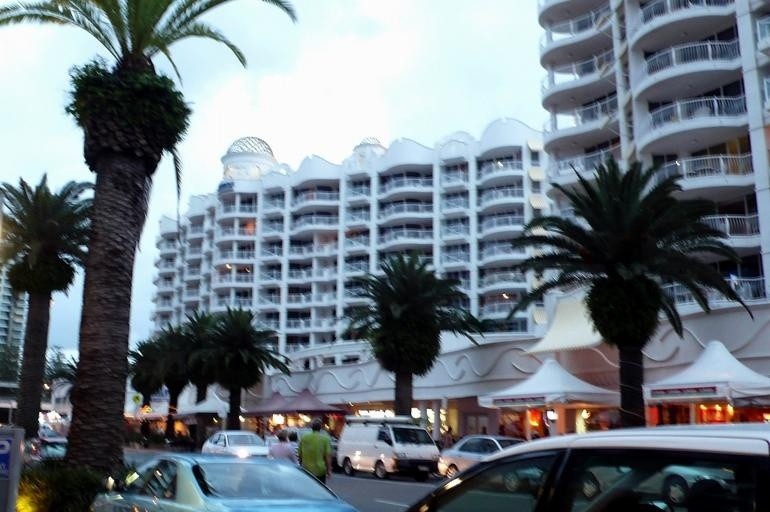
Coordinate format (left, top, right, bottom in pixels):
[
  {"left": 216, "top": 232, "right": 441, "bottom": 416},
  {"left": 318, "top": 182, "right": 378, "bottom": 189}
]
[
  {"left": 582, "top": 462, "right": 739, "bottom": 509},
  {"left": 436, "top": 432, "right": 543, "bottom": 493},
  {"left": 200, "top": 430, "right": 271, "bottom": 460},
  {"left": 403, "top": 423, "right": 770, "bottom": 511},
  {"left": 91, "top": 450, "right": 362, "bottom": 512},
  {"left": 38, "top": 422, "right": 69, "bottom": 461},
  {"left": 272, "top": 426, "right": 343, "bottom": 474}
]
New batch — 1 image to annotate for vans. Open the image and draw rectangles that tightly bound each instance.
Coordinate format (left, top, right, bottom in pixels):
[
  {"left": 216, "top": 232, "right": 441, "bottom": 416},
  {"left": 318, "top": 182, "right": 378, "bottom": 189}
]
[{"left": 337, "top": 413, "right": 439, "bottom": 483}]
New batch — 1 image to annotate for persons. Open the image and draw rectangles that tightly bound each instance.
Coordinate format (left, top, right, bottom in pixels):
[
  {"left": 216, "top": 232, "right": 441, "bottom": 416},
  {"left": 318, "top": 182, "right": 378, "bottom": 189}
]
[
  {"left": 288, "top": 431, "right": 300, "bottom": 448},
  {"left": 497, "top": 424, "right": 506, "bottom": 434},
  {"left": 481, "top": 425, "right": 489, "bottom": 434},
  {"left": 297, "top": 416, "right": 333, "bottom": 488},
  {"left": 268, "top": 430, "right": 297, "bottom": 464},
  {"left": 441, "top": 425, "right": 454, "bottom": 448}
]
